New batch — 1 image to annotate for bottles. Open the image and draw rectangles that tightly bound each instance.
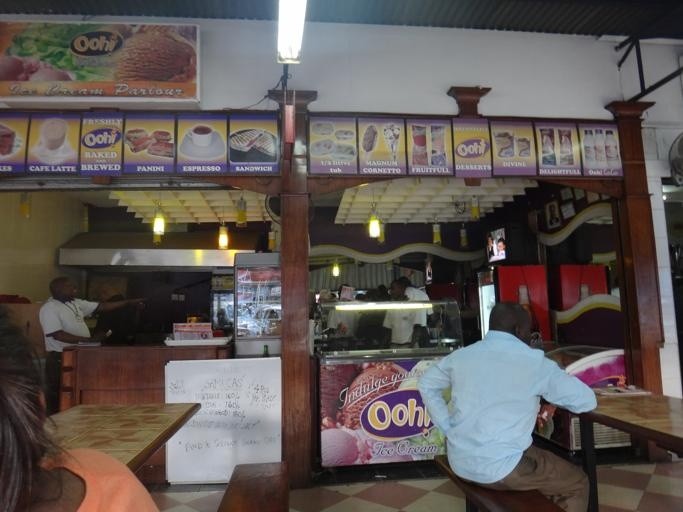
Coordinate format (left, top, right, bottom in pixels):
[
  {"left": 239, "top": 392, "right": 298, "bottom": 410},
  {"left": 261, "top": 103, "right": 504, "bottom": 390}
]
[{"left": 516, "top": 283, "right": 543, "bottom": 348}]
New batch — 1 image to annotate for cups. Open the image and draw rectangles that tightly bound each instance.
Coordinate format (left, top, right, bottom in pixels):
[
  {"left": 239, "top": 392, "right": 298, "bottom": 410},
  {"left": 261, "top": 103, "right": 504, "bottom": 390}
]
[
  {"left": 36, "top": 119, "right": 68, "bottom": 151},
  {"left": 188, "top": 124, "right": 214, "bottom": 148}
]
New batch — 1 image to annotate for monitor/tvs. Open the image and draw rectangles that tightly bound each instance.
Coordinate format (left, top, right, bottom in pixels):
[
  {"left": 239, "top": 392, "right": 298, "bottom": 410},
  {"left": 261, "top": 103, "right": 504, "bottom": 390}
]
[
  {"left": 210, "top": 289, "right": 234, "bottom": 329},
  {"left": 484, "top": 224, "right": 511, "bottom": 265}
]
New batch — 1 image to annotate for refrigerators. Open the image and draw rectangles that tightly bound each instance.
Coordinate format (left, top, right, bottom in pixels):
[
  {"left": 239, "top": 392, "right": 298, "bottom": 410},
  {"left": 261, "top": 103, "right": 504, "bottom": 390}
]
[
  {"left": 532, "top": 343, "right": 632, "bottom": 459},
  {"left": 475, "top": 264, "right": 554, "bottom": 353}
]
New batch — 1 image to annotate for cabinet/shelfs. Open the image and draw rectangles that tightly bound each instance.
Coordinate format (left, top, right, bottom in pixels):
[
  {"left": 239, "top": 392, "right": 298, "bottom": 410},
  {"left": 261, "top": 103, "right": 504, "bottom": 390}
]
[{"left": 312, "top": 297, "right": 465, "bottom": 479}]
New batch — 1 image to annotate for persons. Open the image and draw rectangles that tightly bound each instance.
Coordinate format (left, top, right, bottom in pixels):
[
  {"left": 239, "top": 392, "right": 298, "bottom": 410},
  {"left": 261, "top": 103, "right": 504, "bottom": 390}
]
[
  {"left": 306, "top": 274, "right": 436, "bottom": 350},
  {"left": 0, "top": 297, "right": 159, "bottom": 512},
  {"left": 497, "top": 235, "right": 506, "bottom": 260},
  {"left": 417, "top": 301, "right": 599, "bottom": 511},
  {"left": 487, "top": 233, "right": 497, "bottom": 258},
  {"left": 39, "top": 278, "right": 148, "bottom": 416},
  {"left": 548, "top": 204, "right": 559, "bottom": 225}
]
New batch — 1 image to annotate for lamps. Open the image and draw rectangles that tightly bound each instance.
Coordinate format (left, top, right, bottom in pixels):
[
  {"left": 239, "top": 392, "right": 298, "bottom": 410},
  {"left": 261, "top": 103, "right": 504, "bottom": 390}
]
[
  {"left": 432, "top": 194, "right": 480, "bottom": 248},
  {"left": 151, "top": 189, "right": 165, "bottom": 244},
  {"left": 332, "top": 257, "right": 339, "bottom": 277},
  {"left": 276, "top": 0, "right": 307, "bottom": 66},
  {"left": 367, "top": 187, "right": 385, "bottom": 244},
  {"left": 217, "top": 189, "right": 248, "bottom": 250}
]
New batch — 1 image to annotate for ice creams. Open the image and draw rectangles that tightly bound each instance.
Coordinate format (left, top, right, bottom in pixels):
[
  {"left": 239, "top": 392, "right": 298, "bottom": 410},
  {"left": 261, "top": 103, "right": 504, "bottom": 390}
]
[{"left": 383, "top": 123, "right": 401, "bottom": 161}]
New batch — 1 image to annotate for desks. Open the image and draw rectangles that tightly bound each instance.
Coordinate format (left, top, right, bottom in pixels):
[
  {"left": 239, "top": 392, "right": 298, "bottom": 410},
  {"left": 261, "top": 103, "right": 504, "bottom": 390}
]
[
  {"left": 577, "top": 386, "right": 683, "bottom": 512},
  {"left": 41, "top": 402, "right": 201, "bottom": 474}
]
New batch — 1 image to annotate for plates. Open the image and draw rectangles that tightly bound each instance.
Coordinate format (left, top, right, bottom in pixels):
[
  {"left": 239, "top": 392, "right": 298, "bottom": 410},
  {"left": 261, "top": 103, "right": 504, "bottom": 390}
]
[
  {"left": 181, "top": 132, "right": 226, "bottom": 162},
  {"left": 30, "top": 145, "right": 73, "bottom": 165}
]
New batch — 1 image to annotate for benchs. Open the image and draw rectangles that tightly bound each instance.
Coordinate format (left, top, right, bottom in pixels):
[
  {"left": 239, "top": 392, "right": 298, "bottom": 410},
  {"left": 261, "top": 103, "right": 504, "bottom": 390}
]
[
  {"left": 216, "top": 460, "right": 289, "bottom": 512},
  {"left": 433, "top": 454, "right": 566, "bottom": 512}
]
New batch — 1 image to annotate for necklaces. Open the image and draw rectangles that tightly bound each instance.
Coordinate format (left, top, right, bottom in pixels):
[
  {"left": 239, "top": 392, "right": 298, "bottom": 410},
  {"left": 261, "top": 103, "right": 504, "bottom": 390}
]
[{"left": 64, "top": 301, "right": 79, "bottom": 316}]
